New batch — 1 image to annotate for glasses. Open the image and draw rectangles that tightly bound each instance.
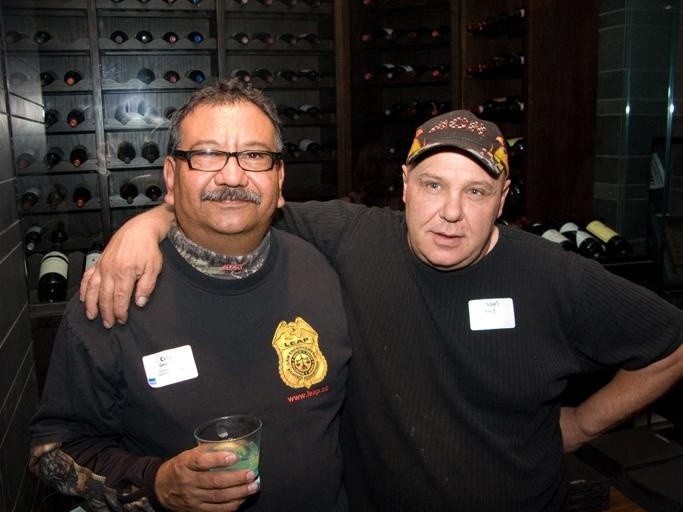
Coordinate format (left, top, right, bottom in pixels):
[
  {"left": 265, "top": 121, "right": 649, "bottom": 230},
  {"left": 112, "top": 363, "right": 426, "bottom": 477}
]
[{"left": 174, "top": 148, "right": 282, "bottom": 172}]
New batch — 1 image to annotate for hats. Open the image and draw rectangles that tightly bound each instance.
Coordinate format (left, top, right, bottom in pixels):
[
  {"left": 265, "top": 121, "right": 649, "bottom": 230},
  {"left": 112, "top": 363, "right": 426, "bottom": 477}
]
[{"left": 407, "top": 109, "right": 509, "bottom": 179}]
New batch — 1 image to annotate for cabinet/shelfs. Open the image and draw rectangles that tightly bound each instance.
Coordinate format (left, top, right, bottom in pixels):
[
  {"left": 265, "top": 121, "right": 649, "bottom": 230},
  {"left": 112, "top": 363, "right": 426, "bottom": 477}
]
[
  {"left": 343, "top": 0, "right": 460, "bottom": 206},
  {"left": 0, "top": 0, "right": 110, "bottom": 320},
  {"left": 459, "top": 2, "right": 599, "bottom": 224},
  {"left": 221, "top": 2, "right": 342, "bottom": 203},
  {"left": 91, "top": 1, "right": 221, "bottom": 213}
]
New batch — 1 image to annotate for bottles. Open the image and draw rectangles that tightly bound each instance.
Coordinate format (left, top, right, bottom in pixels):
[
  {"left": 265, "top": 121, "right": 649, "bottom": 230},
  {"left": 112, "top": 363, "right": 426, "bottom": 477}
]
[
  {"left": 357, "top": 0, "right": 451, "bottom": 199},
  {"left": 462, "top": 6, "right": 527, "bottom": 217},
  {"left": 81, "top": 240, "right": 103, "bottom": 278},
  {"left": 499, "top": 211, "right": 632, "bottom": 263},
  {"left": 227, "top": 0, "right": 328, "bottom": 159},
  {"left": 108, "top": 0, "right": 206, "bottom": 204},
  {"left": 36, "top": 244, "right": 70, "bottom": 304},
  {"left": 2, "top": 29, "right": 91, "bottom": 253}
]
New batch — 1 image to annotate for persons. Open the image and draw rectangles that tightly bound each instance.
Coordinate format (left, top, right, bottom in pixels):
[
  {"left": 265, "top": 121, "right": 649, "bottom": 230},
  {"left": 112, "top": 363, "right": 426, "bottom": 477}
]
[
  {"left": 79, "top": 108, "right": 681, "bottom": 510},
  {"left": 26, "top": 80, "right": 352, "bottom": 512}
]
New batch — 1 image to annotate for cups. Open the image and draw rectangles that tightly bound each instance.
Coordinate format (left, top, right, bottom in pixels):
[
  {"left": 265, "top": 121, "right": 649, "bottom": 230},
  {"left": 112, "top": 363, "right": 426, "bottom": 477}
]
[{"left": 192, "top": 414, "right": 264, "bottom": 493}]
